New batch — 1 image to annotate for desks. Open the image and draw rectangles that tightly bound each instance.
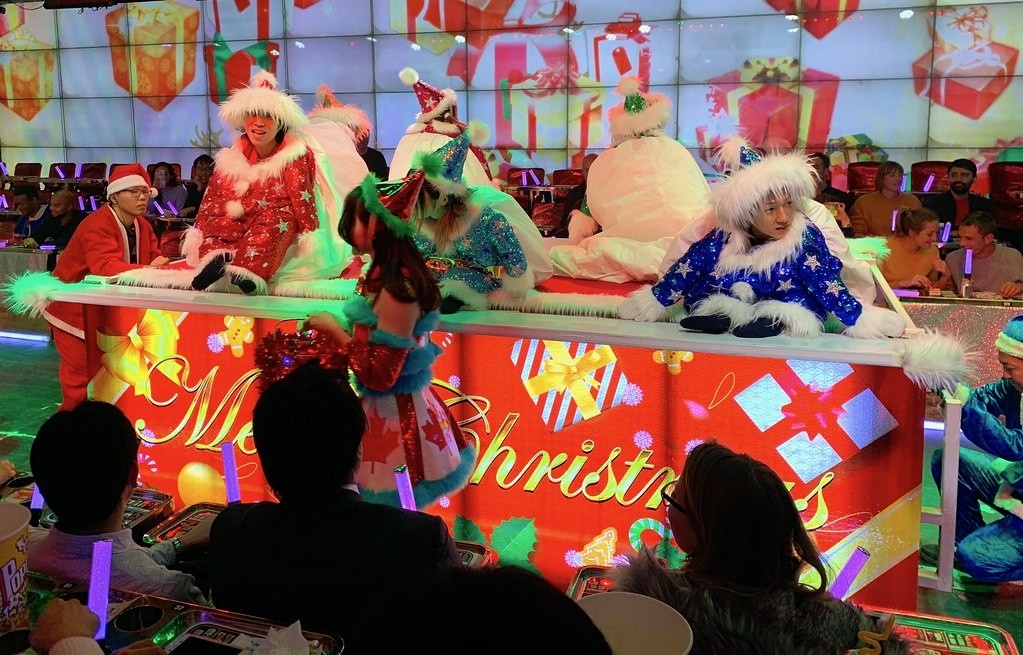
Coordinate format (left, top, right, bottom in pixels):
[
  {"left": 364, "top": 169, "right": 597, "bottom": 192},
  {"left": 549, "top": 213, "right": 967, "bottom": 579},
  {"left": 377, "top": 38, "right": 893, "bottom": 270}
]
[{"left": 893, "top": 285, "right": 1023, "bottom": 412}]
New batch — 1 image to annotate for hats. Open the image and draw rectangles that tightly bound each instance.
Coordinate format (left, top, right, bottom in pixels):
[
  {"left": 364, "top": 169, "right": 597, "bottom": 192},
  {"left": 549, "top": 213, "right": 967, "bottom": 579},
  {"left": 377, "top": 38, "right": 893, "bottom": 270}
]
[
  {"left": 106, "top": 162, "right": 156, "bottom": 199},
  {"left": 714, "top": 137, "right": 818, "bottom": 226},
  {"left": 362, "top": 151, "right": 436, "bottom": 233},
  {"left": 399, "top": 67, "right": 457, "bottom": 120},
  {"left": 993, "top": 314, "right": 1023, "bottom": 360},
  {"left": 212, "top": 71, "right": 306, "bottom": 133},
  {"left": 418, "top": 121, "right": 487, "bottom": 194},
  {"left": 310, "top": 87, "right": 370, "bottom": 140},
  {"left": 602, "top": 74, "right": 667, "bottom": 134}
]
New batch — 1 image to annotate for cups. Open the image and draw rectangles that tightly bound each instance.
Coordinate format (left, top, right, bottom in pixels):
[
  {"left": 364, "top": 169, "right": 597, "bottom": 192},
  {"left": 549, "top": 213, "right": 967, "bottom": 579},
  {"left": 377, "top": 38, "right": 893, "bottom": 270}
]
[
  {"left": 0, "top": 501, "right": 32, "bottom": 634},
  {"left": 114, "top": 605, "right": 166, "bottom": 643},
  {"left": 575, "top": 591, "right": 694, "bottom": 654}
]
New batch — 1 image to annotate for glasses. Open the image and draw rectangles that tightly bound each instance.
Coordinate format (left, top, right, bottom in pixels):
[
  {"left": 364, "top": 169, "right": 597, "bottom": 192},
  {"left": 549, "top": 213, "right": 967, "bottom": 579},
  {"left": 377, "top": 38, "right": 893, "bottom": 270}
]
[
  {"left": 657, "top": 481, "right": 694, "bottom": 527},
  {"left": 122, "top": 186, "right": 154, "bottom": 197}
]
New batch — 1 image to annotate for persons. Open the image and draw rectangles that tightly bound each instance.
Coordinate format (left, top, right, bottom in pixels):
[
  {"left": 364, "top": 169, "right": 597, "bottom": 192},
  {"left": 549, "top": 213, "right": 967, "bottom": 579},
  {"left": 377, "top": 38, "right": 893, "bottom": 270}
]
[
  {"left": 612, "top": 137, "right": 911, "bottom": 340},
  {"left": 181, "top": 65, "right": 330, "bottom": 293},
  {"left": 921, "top": 308, "right": 1022, "bottom": 587},
  {"left": 18, "top": 397, "right": 215, "bottom": 611},
  {"left": 869, "top": 205, "right": 951, "bottom": 297},
  {"left": 21, "top": 190, "right": 91, "bottom": 251},
  {"left": 0, "top": 459, "right": 17, "bottom": 488},
  {"left": 567, "top": 75, "right": 713, "bottom": 242},
  {"left": 10, "top": 183, "right": 54, "bottom": 247},
  {"left": 38, "top": 162, "right": 169, "bottom": 415},
  {"left": 146, "top": 161, "right": 188, "bottom": 217},
  {"left": 923, "top": 157, "right": 993, "bottom": 243},
  {"left": 175, "top": 153, "right": 217, "bottom": 219},
  {"left": 404, "top": 168, "right": 451, "bottom": 222},
  {"left": 611, "top": 440, "right": 916, "bottom": 654},
  {"left": 560, "top": 152, "right": 600, "bottom": 231},
  {"left": 353, "top": 125, "right": 390, "bottom": 181},
  {"left": 301, "top": 77, "right": 374, "bottom": 213},
  {"left": 387, "top": 90, "right": 503, "bottom": 195},
  {"left": 29, "top": 597, "right": 106, "bottom": 655},
  {"left": 931, "top": 212, "right": 1022, "bottom": 303},
  {"left": 298, "top": 181, "right": 477, "bottom": 513},
  {"left": 803, "top": 151, "right": 856, "bottom": 229},
  {"left": 198, "top": 357, "right": 464, "bottom": 649},
  {"left": 850, "top": 160, "right": 924, "bottom": 240}
]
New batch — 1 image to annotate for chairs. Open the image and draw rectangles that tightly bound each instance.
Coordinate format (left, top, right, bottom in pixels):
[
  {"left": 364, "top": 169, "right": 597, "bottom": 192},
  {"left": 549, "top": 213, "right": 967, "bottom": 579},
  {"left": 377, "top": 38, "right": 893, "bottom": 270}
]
[
  {"left": 845, "top": 159, "right": 1023, "bottom": 241},
  {"left": 506, "top": 166, "right": 586, "bottom": 231},
  {"left": 10, "top": 160, "right": 184, "bottom": 206}
]
